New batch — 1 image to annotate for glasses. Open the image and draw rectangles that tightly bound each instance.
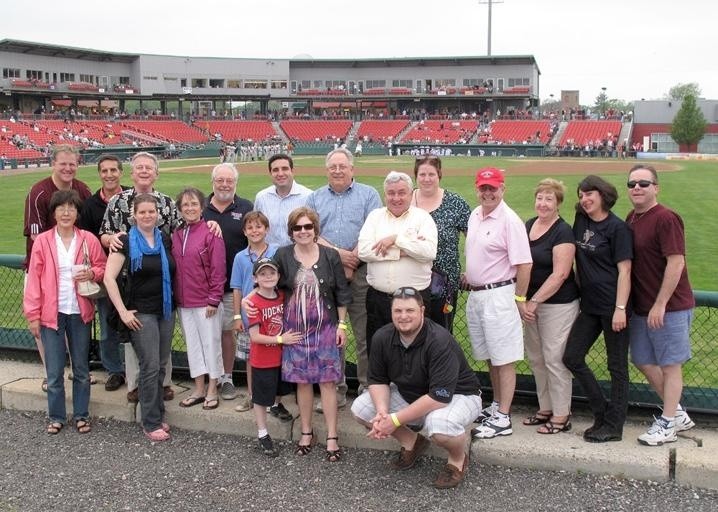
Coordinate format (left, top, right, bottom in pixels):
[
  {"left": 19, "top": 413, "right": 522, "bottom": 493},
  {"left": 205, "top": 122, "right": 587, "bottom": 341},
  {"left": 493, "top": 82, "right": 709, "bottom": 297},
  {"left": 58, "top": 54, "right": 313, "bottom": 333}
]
[
  {"left": 626, "top": 180, "right": 658, "bottom": 189},
  {"left": 478, "top": 187, "right": 499, "bottom": 193},
  {"left": 414, "top": 154, "right": 441, "bottom": 172},
  {"left": 391, "top": 286, "right": 418, "bottom": 297},
  {"left": 291, "top": 223, "right": 314, "bottom": 231}
]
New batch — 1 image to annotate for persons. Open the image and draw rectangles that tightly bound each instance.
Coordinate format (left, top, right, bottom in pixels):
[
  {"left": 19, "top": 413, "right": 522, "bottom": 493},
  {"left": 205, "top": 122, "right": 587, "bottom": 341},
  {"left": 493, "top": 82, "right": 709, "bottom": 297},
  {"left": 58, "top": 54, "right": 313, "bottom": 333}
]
[
  {"left": 22, "top": 145, "right": 93, "bottom": 395},
  {"left": 247, "top": 257, "right": 304, "bottom": 456},
  {"left": 561, "top": 176, "right": 635, "bottom": 442},
  {"left": 1, "top": 101, "right": 215, "bottom": 151},
  {"left": 231, "top": 210, "right": 276, "bottom": 411},
  {"left": 465, "top": 168, "right": 532, "bottom": 438},
  {"left": 625, "top": 166, "right": 695, "bottom": 446},
  {"left": 530, "top": 107, "right": 635, "bottom": 138},
  {"left": 103, "top": 196, "right": 177, "bottom": 440},
  {"left": 79, "top": 154, "right": 140, "bottom": 391},
  {"left": 424, "top": 84, "right": 501, "bottom": 97},
  {"left": 243, "top": 207, "right": 349, "bottom": 462},
  {"left": 316, "top": 135, "right": 395, "bottom": 160},
  {"left": 359, "top": 171, "right": 439, "bottom": 433},
  {"left": 93, "top": 82, "right": 138, "bottom": 95},
  {"left": 522, "top": 177, "right": 580, "bottom": 434},
  {"left": 199, "top": 164, "right": 255, "bottom": 397},
  {"left": 99, "top": 153, "right": 221, "bottom": 403},
  {"left": 251, "top": 154, "right": 314, "bottom": 246},
  {"left": 324, "top": 84, "right": 363, "bottom": 95},
  {"left": 396, "top": 107, "right": 531, "bottom": 137},
  {"left": 213, "top": 132, "right": 300, "bottom": 161},
  {"left": 22, "top": 192, "right": 107, "bottom": 436},
  {"left": 210, "top": 107, "right": 254, "bottom": 118},
  {"left": 396, "top": 138, "right": 643, "bottom": 160},
  {"left": 253, "top": 105, "right": 398, "bottom": 124},
  {"left": 404, "top": 155, "right": 473, "bottom": 336},
  {"left": 26, "top": 76, "right": 52, "bottom": 88},
  {"left": 172, "top": 187, "right": 226, "bottom": 411},
  {"left": 350, "top": 287, "right": 482, "bottom": 489},
  {"left": 305, "top": 149, "right": 385, "bottom": 413}
]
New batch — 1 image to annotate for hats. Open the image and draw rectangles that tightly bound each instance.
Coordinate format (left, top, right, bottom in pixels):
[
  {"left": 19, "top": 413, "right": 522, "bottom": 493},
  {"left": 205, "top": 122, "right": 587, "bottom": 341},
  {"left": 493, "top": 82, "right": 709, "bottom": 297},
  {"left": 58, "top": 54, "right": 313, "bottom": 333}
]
[
  {"left": 475, "top": 167, "right": 504, "bottom": 188},
  {"left": 252, "top": 257, "right": 281, "bottom": 276}
]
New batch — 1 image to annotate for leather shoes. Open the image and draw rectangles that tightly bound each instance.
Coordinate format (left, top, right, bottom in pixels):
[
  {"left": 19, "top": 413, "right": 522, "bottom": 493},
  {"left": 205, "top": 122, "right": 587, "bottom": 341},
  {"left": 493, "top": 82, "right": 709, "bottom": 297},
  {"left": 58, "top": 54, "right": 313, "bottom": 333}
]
[
  {"left": 433, "top": 451, "right": 469, "bottom": 488},
  {"left": 583, "top": 420, "right": 603, "bottom": 435},
  {"left": 583, "top": 424, "right": 623, "bottom": 443},
  {"left": 395, "top": 433, "right": 428, "bottom": 473}
]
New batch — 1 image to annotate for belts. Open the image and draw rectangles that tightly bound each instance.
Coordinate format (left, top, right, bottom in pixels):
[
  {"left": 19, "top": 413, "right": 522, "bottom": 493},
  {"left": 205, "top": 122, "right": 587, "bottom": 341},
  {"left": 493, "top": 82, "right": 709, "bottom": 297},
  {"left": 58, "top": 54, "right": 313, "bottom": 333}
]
[{"left": 464, "top": 278, "right": 517, "bottom": 293}]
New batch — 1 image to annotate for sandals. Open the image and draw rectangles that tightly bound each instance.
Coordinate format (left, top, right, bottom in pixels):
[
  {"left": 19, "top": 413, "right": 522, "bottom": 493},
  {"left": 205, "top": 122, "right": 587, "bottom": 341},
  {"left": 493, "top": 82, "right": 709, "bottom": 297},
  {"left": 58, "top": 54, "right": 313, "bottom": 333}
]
[
  {"left": 76, "top": 418, "right": 92, "bottom": 434},
  {"left": 294, "top": 429, "right": 314, "bottom": 457},
  {"left": 537, "top": 418, "right": 572, "bottom": 435},
  {"left": 325, "top": 434, "right": 342, "bottom": 464},
  {"left": 523, "top": 410, "right": 553, "bottom": 425},
  {"left": 46, "top": 422, "right": 63, "bottom": 435}
]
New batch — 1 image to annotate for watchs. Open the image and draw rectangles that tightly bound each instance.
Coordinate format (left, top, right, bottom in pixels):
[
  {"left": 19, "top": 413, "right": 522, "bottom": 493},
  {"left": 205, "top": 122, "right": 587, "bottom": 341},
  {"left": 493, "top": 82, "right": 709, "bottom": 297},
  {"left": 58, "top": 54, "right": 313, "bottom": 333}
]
[
  {"left": 530, "top": 298, "right": 540, "bottom": 303},
  {"left": 616, "top": 305, "right": 627, "bottom": 310}
]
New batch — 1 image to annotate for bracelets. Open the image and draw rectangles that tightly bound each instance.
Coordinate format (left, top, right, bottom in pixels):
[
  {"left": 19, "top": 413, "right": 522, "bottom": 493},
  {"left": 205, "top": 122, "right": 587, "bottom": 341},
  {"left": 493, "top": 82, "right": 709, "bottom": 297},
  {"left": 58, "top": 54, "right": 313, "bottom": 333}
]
[
  {"left": 231, "top": 315, "right": 241, "bottom": 321},
  {"left": 275, "top": 336, "right": 283, "bottom": 348},
  {"left": 514, "top": 295, "right": 526, "bottom": 302},
  {"left": 336, "top": 320, "right": 345, "bottom": 327},
  {"left": 390, "top": 414, "right": 400, "bottom": 429}
]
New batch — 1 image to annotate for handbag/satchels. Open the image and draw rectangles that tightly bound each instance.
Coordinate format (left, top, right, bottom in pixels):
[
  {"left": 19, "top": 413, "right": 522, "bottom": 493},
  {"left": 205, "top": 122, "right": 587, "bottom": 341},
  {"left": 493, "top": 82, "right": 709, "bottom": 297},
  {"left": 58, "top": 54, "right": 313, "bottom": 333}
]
[{"left": 72, "top": 237, "right": 107, "bottom": 300}]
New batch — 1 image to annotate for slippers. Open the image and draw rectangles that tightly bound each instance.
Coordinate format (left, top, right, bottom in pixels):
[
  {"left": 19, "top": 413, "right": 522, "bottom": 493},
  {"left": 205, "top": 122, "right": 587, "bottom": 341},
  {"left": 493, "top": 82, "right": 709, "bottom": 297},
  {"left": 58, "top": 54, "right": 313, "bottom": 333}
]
[
  {"left": 202, "top": 396, "right": 220, "bottom": 410},
  {"left": 68, "top": 372, "right": 98, "bottom": 384},
  {"left": 41, "top": 377, "right": 50, "bottom": 393},
  {"left": 178, "top": 395, "right": 206, "bottom": 408}
]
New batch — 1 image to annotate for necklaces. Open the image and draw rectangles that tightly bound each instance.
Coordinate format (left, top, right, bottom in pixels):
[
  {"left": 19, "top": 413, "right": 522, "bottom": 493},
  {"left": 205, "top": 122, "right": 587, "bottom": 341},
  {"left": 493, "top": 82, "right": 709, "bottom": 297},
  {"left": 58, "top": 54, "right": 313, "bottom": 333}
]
[{"left": 629, "top": 206, "right": 656, "bottom": 223}]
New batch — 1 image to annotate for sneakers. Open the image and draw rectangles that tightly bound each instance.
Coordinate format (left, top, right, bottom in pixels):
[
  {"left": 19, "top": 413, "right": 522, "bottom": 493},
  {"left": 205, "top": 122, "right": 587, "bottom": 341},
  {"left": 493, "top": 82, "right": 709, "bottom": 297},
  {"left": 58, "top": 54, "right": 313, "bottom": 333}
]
[
  {"left": 258, "top": 433, "right": 279, "bottom": 458},
  {"left": 315, "top": 396, "right": 347, "bottom": 414},
  {"left": 127, "top": 387, "right": 139, "bottom": 402},
  {"left": 472, "top": 404, "right": 499, "bottom": 424},
  {"left": 105, "top": 375, "right": 125, "bottom": 391},
  {"left": 671, "top": 409, "right": 696, "bottom": 435},
  {"left": 163, "top": 386, "right": 175, "bottom": 401},
  {"left": 142, "top": 428, "right": 170, "bottom": 441},
  {"left": 161, "top": 423, "right": 171, "bottom": 433},
  {"left": 470, "top": 409, "right": 513, "bottom": 439},
  {"left": 234, "top": 399, "right": 254, "bottom": 413},
  {"left": 270, "top": 402, "right": 294, "bottom": 423},
  {"left": 222, "top": 381, "right": 237, "bottom": 400},
  {"left": 636, "top": 413, "right": 680, "bottom": 446}
]
